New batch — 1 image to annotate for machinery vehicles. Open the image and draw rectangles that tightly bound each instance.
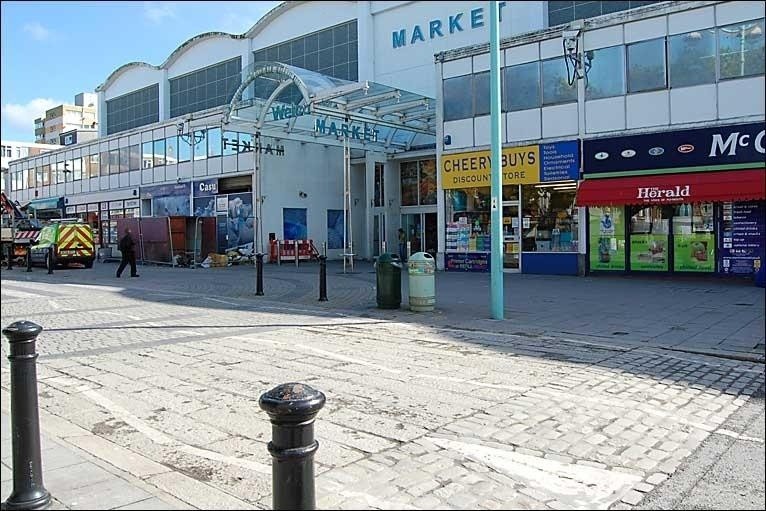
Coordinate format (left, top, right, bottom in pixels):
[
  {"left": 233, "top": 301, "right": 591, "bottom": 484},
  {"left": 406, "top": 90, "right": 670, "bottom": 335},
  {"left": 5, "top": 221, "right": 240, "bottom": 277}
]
[{"left": 1, "top": 190, "right": 44, "bottom": 266}]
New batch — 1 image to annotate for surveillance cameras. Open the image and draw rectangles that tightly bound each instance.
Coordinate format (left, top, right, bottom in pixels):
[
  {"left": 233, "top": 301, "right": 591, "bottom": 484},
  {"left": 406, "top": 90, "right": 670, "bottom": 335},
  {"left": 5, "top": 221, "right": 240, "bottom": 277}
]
[
  {"left": 176, "top": 122, "right": 184, "bottom": 132},
  {"left": 562, "top": 30, "right": 582, "bottom": 48}
]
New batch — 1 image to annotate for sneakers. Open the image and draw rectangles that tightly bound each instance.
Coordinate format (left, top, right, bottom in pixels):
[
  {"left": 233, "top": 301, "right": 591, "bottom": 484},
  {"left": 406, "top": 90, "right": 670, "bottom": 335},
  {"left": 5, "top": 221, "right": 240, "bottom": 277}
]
[
  {"left": 116, "top": 272, "right": 121, "bottom": 278},
  {"left": 131, "top": 273, "right": 140, "bottom": 276}
]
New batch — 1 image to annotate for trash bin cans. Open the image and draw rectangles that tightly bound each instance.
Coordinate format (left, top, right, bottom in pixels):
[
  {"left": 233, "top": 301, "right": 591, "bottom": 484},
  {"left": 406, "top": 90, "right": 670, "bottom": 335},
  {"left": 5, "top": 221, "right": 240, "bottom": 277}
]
[
  {"left": 407, "top": 252, "right": 436, "bottom": 312},
  {"left": 375, "top": 252, "right": 403, "bottom": 309}
]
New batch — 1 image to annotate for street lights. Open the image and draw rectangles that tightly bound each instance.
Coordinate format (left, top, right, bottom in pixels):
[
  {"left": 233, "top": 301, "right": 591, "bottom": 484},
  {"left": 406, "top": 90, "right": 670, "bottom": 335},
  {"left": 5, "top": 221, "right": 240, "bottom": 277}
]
[{"left": 177, "top": 122, "right": 207, "bottom": 217}]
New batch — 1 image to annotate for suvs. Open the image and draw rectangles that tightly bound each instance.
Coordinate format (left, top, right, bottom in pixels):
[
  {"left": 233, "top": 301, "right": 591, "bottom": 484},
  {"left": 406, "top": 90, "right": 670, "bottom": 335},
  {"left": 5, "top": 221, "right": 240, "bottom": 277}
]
[{"left": 26, "top": 217, "right": 97, "bottom": 270}]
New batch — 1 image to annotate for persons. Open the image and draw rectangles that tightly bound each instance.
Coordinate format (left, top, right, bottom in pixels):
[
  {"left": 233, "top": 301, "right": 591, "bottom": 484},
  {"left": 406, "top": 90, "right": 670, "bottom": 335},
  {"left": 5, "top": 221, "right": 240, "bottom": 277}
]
[
  {"left": 116, "top": 228, "right": 139, "bottom": 278},
  {"left": 398, "top": 228, "right": 407, "bottom": 261}
]
